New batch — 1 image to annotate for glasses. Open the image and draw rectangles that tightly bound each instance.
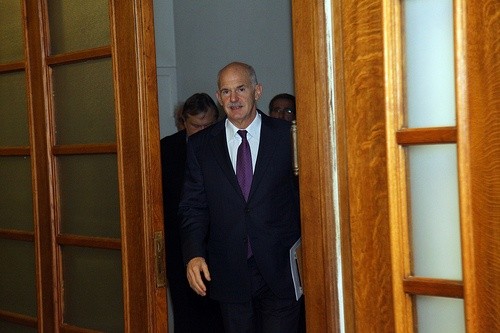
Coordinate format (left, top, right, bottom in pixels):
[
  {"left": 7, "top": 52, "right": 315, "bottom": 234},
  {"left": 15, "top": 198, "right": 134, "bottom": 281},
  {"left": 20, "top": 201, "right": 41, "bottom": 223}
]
[{"left": 272, "top": 107, "right": 293, "bottom": 113}]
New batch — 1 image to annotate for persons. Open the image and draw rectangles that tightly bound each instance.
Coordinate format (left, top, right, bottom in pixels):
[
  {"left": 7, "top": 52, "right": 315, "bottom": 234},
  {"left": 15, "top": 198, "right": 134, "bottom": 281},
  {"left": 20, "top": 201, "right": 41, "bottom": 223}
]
[
  {"left": 159, "top": 92, "right": 220, "bottom": 333},
  {"left": 179, "top": 61, "right": 304, "bottom": 333}
]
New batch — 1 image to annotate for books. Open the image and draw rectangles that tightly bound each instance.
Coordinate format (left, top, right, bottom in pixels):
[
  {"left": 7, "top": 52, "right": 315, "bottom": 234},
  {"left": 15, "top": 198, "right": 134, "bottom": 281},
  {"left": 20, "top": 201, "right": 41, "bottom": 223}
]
[{"left": 290, "top": 236, "right": 303, "bottom": 301}]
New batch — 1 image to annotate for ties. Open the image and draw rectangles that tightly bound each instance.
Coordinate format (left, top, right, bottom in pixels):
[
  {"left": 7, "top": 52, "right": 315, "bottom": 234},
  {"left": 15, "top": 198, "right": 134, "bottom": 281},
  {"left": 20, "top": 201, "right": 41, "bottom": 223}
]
[{"left": 236, "top": 130, "right": 253, "bottom": 259}]
